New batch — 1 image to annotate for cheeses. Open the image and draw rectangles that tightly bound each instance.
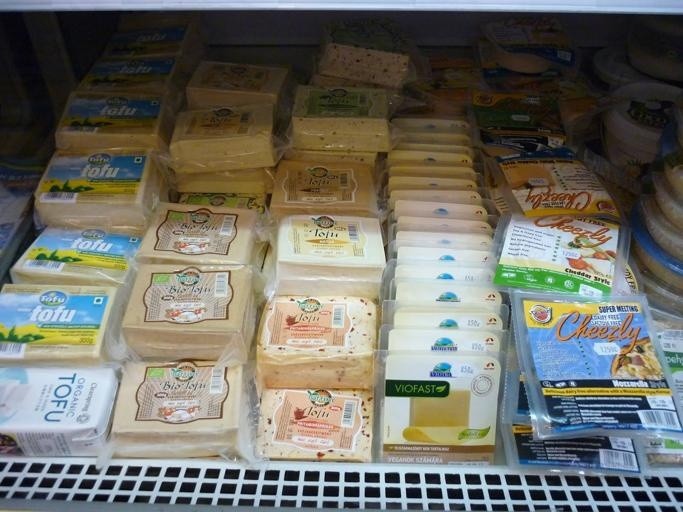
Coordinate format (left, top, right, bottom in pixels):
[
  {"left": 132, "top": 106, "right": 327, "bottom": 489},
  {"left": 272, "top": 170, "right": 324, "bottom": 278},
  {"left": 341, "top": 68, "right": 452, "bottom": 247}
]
[{"left": 402, "top": 391, "right": 494, "bottom": 445}]
